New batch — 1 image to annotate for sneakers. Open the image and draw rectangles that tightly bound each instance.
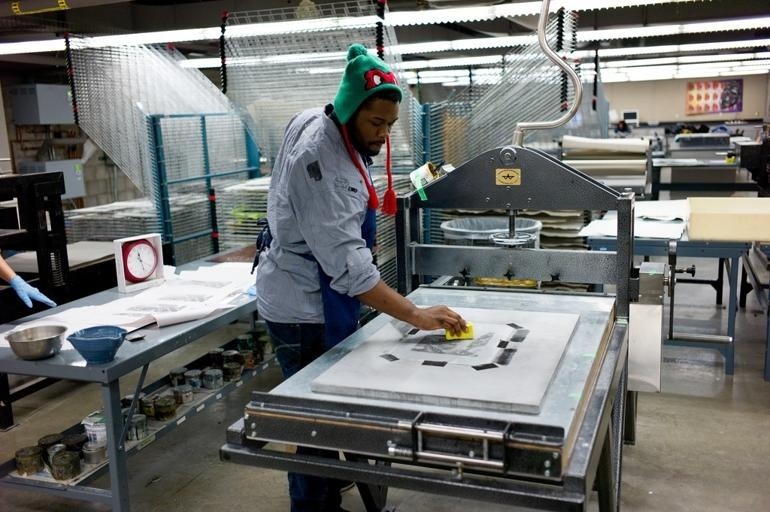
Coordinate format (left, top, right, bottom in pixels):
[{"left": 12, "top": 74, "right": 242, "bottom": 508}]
[{"left": 337, "top": 479, "right": 356, "bottom": 492}]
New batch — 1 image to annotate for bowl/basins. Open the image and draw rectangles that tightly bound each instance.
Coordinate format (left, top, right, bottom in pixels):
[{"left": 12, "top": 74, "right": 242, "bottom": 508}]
[
  {"left": 66, "top": 325, "right": 128, "bottom": 365},
  {"left": 4, "top": 325, "right": 69, "bottom": 361}
]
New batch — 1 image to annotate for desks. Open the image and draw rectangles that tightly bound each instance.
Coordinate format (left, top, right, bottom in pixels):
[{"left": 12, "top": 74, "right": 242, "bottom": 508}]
[{"left": 0, "top": 247, "right": 256, "bottom": 512}]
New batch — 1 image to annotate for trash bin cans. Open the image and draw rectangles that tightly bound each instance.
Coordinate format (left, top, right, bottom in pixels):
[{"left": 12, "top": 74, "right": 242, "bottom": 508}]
[{"left": 440, "top": 216, "right": 542, "bottom": 288}]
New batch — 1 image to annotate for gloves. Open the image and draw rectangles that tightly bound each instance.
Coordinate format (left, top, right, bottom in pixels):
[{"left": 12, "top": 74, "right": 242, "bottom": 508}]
[{"left": 8, "top": 275, "right": 58, "bottom": 308}]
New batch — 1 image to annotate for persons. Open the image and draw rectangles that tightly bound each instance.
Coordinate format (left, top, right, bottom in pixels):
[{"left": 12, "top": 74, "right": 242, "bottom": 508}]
[
  {"left": 615, "top": 120, "right": 632, "bottom": 134},
  {"left": 0, "top": 253, "right": 57, "bottom": 310},
  {"left": 250, "top": 44, "right": 468, "bottom": 512}
]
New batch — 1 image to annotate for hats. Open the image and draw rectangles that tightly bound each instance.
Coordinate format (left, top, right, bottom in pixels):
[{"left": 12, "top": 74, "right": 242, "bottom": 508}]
[{"left": 333, "top": 44, "right": 404, "bottom": 124}]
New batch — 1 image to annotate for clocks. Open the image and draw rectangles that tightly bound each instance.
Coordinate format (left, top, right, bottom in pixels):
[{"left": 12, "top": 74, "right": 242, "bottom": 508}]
[{"left": 113, "top": 233, "right": 166, "bottom": 293}]
